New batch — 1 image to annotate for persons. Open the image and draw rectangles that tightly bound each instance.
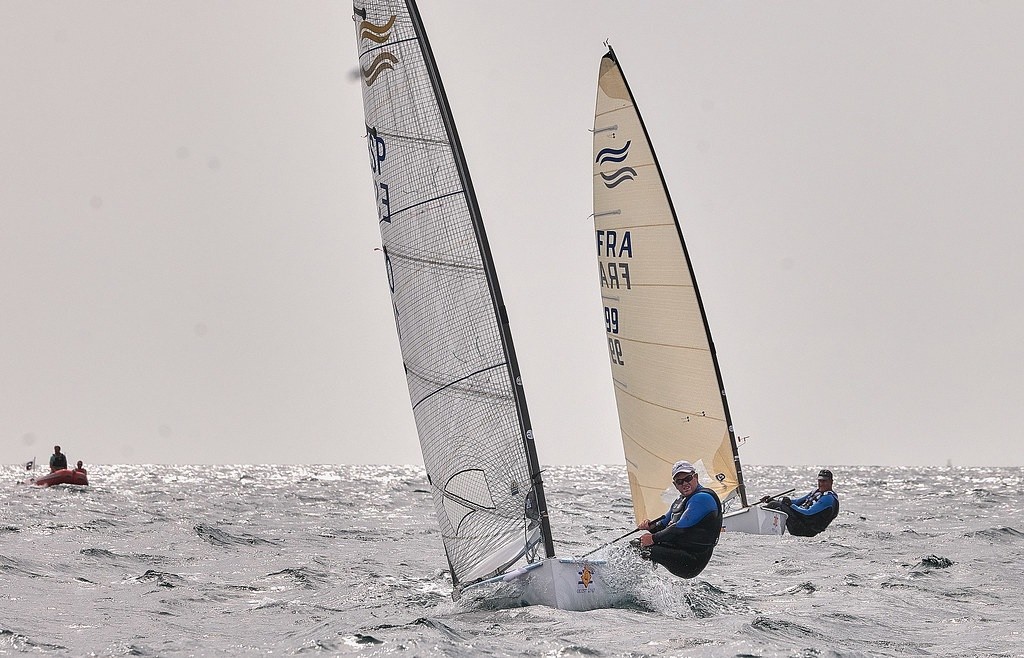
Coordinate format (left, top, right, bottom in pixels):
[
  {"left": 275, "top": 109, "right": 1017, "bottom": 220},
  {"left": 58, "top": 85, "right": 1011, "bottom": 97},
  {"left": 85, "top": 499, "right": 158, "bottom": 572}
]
[
  {"left": 627, "top": 460, "right": 722, "bottom": 580},
  {"left": 49, "top": 446, "right": 67, "bottom": 473},
  {"left": 764, "top": 469, "right": 840, "bottom": 537},
  {"left": 73, "top": 461, "right": 88, "bottom": 475}
]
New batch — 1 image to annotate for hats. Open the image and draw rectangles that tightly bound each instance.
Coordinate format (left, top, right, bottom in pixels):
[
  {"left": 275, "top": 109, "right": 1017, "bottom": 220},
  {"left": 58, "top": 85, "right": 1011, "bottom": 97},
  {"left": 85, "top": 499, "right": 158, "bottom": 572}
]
[
  {"left": 817, "top": 470, "right": 833, "bottom": 481},
  {"left": 671, "top": 460, "right": 696, "bottom": 480}
]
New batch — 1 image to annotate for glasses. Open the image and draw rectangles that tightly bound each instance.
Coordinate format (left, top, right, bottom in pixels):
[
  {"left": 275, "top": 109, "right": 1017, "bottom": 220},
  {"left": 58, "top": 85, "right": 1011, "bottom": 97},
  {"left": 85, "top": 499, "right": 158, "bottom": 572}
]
[{"left": 675, "top": 472, "right": 697, "bottom": 485}]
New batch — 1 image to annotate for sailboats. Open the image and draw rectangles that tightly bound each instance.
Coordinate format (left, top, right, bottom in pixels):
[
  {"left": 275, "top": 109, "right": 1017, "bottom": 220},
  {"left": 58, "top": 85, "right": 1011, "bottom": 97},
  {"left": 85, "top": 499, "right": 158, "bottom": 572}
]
[
  {"left": 589, "top": 44, "right": 787, "bottom": 537},
  {"left": 349, "top": 0, "right": 618, "bottom": 615}
]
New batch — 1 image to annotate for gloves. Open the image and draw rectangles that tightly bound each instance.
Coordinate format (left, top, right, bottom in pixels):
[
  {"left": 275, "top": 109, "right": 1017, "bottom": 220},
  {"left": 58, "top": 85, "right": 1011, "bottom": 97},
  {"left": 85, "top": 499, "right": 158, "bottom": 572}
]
[
  {"left": 760, "top": 495, "right": 776, "bottom": 503},
  {"left": 779, "top": 496, "right": 793, "bottom": 507}
]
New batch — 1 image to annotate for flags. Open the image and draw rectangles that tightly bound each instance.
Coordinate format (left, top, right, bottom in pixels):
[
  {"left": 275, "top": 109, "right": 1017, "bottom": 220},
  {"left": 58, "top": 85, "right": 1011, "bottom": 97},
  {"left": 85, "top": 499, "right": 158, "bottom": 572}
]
[{"left": 24, "top": 458, "right": 35, "bottom": 474}]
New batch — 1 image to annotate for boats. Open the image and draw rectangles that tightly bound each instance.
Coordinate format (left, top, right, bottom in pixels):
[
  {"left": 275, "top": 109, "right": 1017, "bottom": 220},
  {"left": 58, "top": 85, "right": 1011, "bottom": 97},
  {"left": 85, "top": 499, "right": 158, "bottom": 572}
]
[{"left": 36, "top": 469, "right": 88, "bottom": 486}]
